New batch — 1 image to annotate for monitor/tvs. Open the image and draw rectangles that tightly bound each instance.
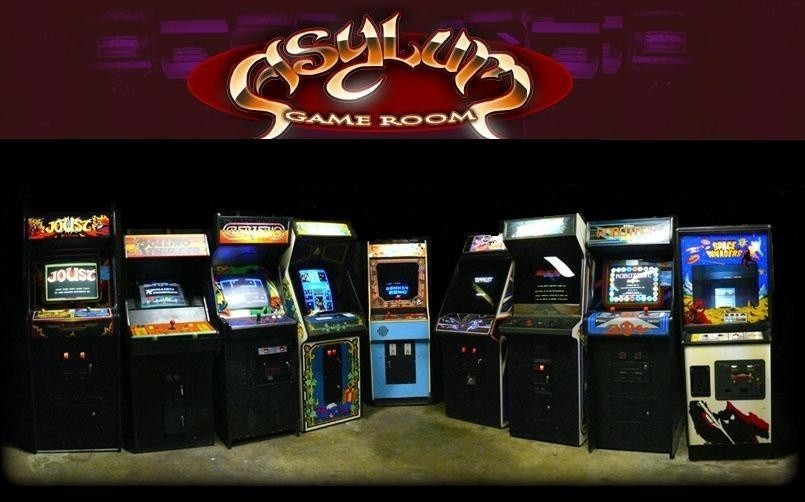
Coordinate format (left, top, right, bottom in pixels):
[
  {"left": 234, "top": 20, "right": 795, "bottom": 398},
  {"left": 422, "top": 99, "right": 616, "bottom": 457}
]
[
  {"left": 601, "top": 258, "right": 663, "bottom": 309},
  {"left": 298, "top": 269, "right": 336, "bottom": 315},
  {"left": 383, "top": 272, "right": 412, "bottom": 297},
  {"left": 533, "top": 264, "right": 569, "bottom": 304},
  {"left": 134, "top": 275, "right": 192, "bottom": 308},
  {"left": 471, "top": 269, "right": 499, "bottom": 298},
  {"left": 39, "top": 257, "right": 102, "bottom": 304},
  {"left": 219, "top": 275, "right": 273, "bottom": 310}
]
